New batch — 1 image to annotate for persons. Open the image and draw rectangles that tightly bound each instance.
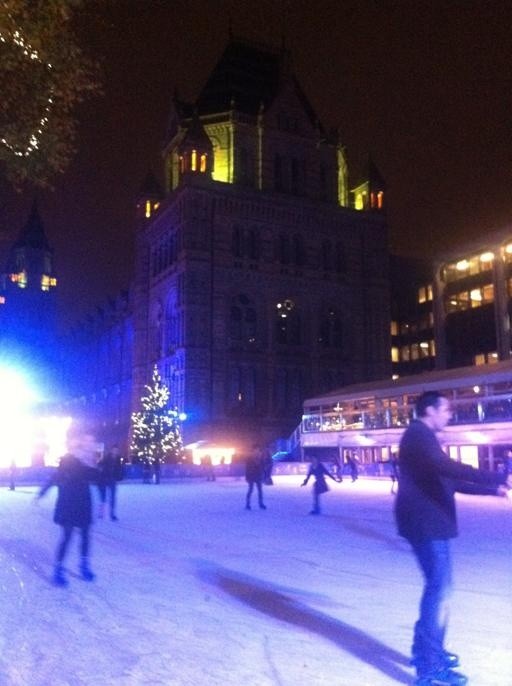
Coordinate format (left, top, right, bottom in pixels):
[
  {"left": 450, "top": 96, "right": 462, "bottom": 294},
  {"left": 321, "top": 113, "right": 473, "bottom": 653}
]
[
  {"left": 153, "top": 460, "right": 161, "bottom": 485},
  {"left": 244, "top": 445, "right": 265, "bottom": 510},
  {"left": 142, "top": 458, "right": 152, "bottom": 484},
  {"left": 343, "top": 455, "right": 358, "bottom": 482},
  {"left": 198, "top": 452, "right": 216, "bottom": 481},
  {"left": 33, "top": 431, "right": 106, "bottom": 588},
  {"left": 300, "top": 454, "right": 337, "bottom": 515},
  {"left": 97, "top": 444, "right": 126, "bottom": 521},
  {"left": 387, "top": 453, "right": 398, "bottom": 482},
  {"left": 394, "top": 388, "right": 511, "bottom": 685},
  {"left": 261, "top": 447, "right": 275, "bottom": 485},
  {"left": 329, "top": 455, "right": 342, "bottom": 482}
]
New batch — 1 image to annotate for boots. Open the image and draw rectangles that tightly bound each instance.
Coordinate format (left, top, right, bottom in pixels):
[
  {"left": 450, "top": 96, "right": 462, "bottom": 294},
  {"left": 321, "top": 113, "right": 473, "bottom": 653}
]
[
  {"left": 83, "top": 557, "right": 97, "bottom": 580},
  {"left": 54, "top": 562, "right": 69, "bottom": 587}
]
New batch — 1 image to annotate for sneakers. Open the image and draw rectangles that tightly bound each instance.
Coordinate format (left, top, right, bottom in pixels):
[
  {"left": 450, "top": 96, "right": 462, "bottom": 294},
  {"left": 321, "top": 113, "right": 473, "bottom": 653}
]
[
  {"left": 259, "top": 504, "right": 266, "bottom": 508},
  {"left": 445, "top": 651, "right": 457, "bottom": 664},
  {"left": 109, "top": 515, "right": 117, "bottom": 520},
  {"left": 311, "top": 509, "right": 319, "bottom": 513},
  {"left": 419, "top": 660, "right": 466, "bottom": 686},
  {"left": 247, "top": 505, "right": 251, "bottom": 510}
]
